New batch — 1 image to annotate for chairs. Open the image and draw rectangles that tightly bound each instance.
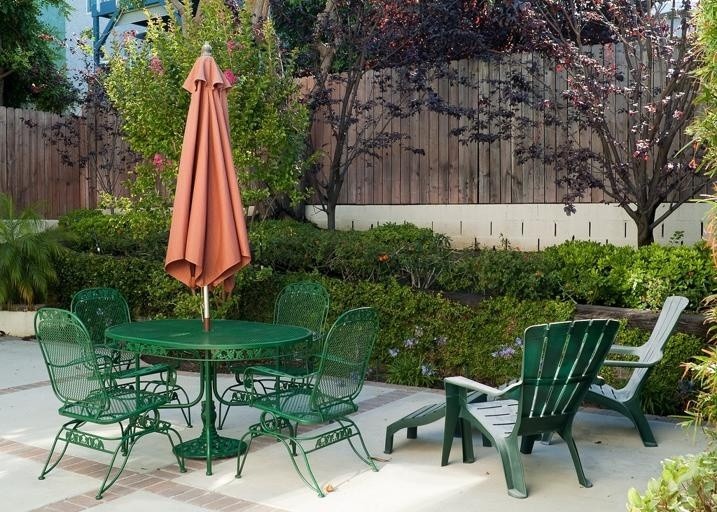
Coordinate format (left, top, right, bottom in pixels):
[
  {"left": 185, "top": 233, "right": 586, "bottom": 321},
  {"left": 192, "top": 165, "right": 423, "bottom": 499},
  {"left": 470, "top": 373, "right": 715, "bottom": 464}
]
[
  {"left": 216, "top": 282, "right": 330, "bottom": 430},
  {"left": 235, "top": 307, "right": 378, "bottom": 497},
  {"left": 385, "top": 295, "right": 688, "bottom": 453},
  {"left": 34, "top": 307, "right": 185, "bottom": 500},
  {"left": 440, "top": 319, "right": 620, "bottom": 498},
  {"left": 70, "top": 286, "right": 193, "bottom": 430}
]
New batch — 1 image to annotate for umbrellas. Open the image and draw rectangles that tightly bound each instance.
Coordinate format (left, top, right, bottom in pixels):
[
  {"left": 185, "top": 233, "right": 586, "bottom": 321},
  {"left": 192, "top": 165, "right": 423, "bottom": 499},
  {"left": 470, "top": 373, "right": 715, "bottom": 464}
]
[{"left": 164, "top": 43, "right": 251, "bottom": 401}]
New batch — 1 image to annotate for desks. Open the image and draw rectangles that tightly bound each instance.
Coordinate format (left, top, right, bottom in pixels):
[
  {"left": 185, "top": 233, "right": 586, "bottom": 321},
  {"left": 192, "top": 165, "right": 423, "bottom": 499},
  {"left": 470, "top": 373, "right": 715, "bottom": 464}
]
[{"left": 104, "top": 318, "right": 312, "bottom": 460}]
